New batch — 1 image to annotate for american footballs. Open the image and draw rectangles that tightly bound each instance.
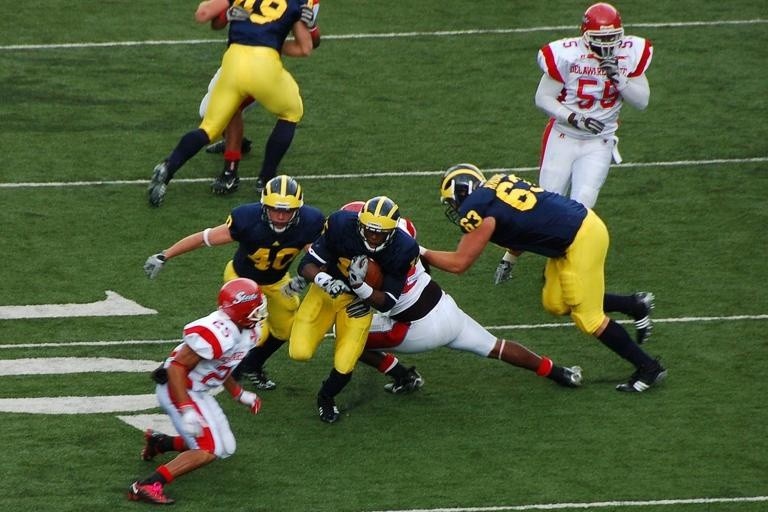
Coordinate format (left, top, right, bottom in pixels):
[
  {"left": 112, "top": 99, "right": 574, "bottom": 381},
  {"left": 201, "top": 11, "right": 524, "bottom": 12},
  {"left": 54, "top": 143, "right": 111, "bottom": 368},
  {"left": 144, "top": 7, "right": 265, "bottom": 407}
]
[{"left": 360, "top": 256, "right": 384, "bottom": 291}]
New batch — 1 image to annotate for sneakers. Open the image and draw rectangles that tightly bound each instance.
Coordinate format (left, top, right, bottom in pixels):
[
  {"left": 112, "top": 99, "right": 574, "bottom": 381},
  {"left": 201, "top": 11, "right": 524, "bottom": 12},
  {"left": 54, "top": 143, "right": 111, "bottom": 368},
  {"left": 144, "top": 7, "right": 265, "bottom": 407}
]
[
  {"left": 316, "top": 392, "right": 340, "bottom": 424},
  {"left": 141, "top": 430, "right": 163, "bottom": 462},
  {"left": 127, "top": 481, "right": 175, "bottom": 505},
  {"left": 613, "top": 356, "right": 667, "bottom": 393},
  {"left": 565, "top": 365, "right": 585, "bottom": 388},
  {"left": 385, "top": 367, "right": 426, "bottom": 393},
  {"left": 632, "top": 292, "right": 656, "bottom": 345},
  {"left": 147, "top": 137, "right": 266, "bottom": 206},
  {"left": 235, "top": 358, "right": 276, "bottom": 390}
]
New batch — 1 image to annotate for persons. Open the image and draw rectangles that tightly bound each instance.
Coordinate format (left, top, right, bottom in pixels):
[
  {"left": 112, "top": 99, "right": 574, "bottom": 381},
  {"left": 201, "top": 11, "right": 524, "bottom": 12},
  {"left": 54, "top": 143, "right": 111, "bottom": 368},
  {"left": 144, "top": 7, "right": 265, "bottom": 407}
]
[
  {"left": 144, "top": 0, "right": 314, "bottom": 210},
  {"left": 200, "top": 1, "right": 322, "bottom": 193},
  {"left": 339, "top": 200, "right": 584, "bottom": 396},
  {"left": 125, "top": 276, "right": 267, "bottom": 506},
  {"left": 141, "top": 175, "right": 327, "bottom": 391},
  {"left": 287, "top": 194, "right": 419, "bottom": 423},
  {"left": 420, "top": 163, "right": 670, "bottom": 393},
  {"left": 534, "top": 2, "right": 656, "bottom": 282}
]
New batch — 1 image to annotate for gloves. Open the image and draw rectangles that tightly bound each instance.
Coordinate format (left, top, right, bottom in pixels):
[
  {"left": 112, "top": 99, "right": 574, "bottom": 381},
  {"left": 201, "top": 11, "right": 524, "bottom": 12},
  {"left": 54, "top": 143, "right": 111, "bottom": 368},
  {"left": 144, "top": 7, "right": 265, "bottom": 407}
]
[
  {"left": 313, "top": 271, "right": 351, "bottom": 297},
  {"left": 348, "top": 255, "right": 368, "bottom": 287},
  {"left": 177, "top": 400, "right": 206, "bottom": 436},
  {"left": 598, "top": 54, "right": 621, "bottom": 83},
  {"left": 494, "top": 259, "right": 513, "bottom": 286},
  {"left": 280, "top": 275, "right": 309, "bottom": 297},
  {"left": 345, "top": 298, "right": 371, "bottom": 318},
  {"left": 143, "top": 249, "right": 166, "bottom": 281},
  {"left": 571, "top": 113, "right": 605, "bottom": 135},
  {"left": 230, "top": 387, "right": 262, "bottom": 414}
]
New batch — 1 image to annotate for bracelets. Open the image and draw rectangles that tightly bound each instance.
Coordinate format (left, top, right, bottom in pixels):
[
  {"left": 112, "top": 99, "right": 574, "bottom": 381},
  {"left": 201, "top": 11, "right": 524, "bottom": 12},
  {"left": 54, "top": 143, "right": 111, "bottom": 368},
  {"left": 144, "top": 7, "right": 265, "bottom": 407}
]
[
  {"left": 314, "top": 270, "right": 334, "bottom": 289},
  {"left": 418, "top": 244, "right": 428, "bottom": 256},
  {"left": 351, "top": 282, "right": 375, "bottom": 299},
  {"left": 503, "top": 250, "right": 519, "bottom": 266}
]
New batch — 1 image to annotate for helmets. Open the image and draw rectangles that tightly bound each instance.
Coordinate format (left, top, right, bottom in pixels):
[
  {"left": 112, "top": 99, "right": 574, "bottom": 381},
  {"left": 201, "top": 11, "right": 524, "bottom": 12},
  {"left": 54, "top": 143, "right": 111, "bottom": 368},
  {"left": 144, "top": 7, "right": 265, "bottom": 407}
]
[
  {"left": 217, "top": 277, "right": 268, "bottom": 330},
  {"left": 356, "top": 195, "right": 400, "bottom": 261},
  {"left": 441, "top": 163, "right": 488, "bottom": 226},
  {"left": 260, "top": 175, "right": 303, "bottom": 236},
  {"left": 580, "top": 2, "right": 625, "bottom": 61}
]
